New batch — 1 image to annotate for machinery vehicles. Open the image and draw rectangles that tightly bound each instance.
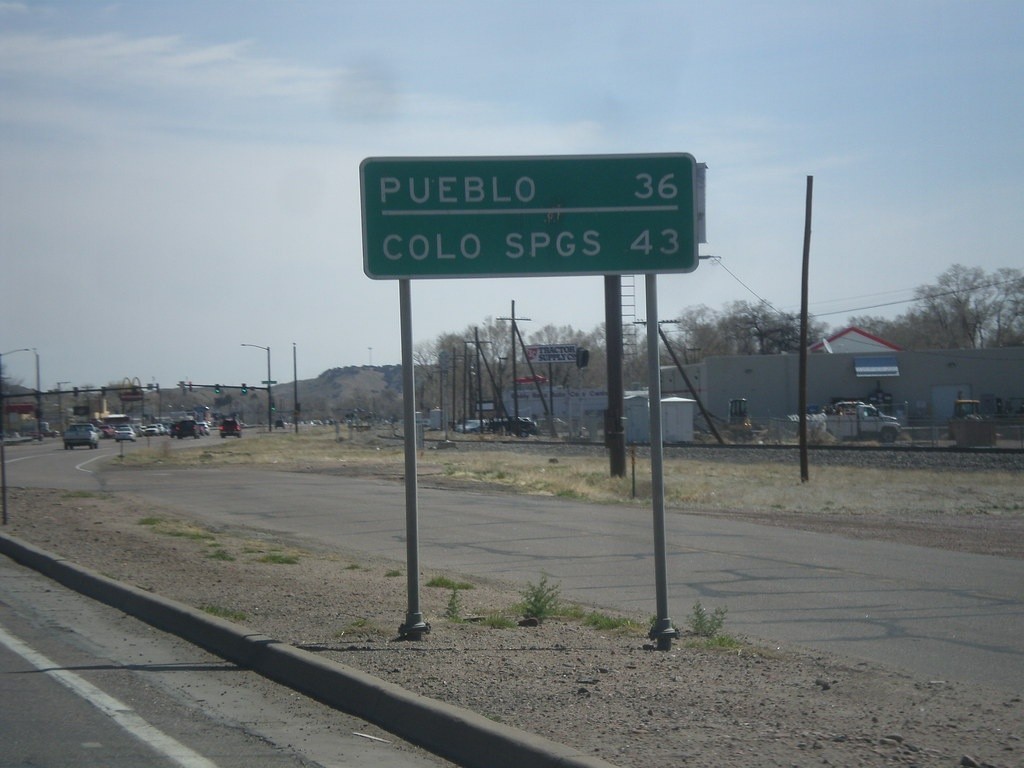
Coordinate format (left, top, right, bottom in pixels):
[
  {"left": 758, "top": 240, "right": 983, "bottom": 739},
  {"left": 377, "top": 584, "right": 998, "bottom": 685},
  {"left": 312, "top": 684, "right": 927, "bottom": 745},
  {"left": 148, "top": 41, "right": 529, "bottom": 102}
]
[
  {"left": 947, "top": 396, "right": 997, "bottom": 448},
  {"left": 725, "top": 397, "right": 752, "bottom": 442}
]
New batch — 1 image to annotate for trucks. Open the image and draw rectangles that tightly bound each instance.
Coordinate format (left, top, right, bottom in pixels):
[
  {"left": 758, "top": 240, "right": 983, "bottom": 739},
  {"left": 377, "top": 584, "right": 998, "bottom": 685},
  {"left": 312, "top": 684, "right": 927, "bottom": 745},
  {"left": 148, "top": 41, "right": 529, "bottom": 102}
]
[{"left": 819, "top": 404, "right": 902, "bottom": 445}]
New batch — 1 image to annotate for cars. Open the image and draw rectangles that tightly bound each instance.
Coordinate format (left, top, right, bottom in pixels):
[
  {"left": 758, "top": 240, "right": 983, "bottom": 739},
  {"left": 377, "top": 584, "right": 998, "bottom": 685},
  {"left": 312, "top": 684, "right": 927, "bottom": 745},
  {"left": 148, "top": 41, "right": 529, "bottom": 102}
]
[
  {"left": 36, "top": 411, "right": 286, "bottom": 450},
  {"left": 455, "top": 414, "right": 567, "bottom": 439}
]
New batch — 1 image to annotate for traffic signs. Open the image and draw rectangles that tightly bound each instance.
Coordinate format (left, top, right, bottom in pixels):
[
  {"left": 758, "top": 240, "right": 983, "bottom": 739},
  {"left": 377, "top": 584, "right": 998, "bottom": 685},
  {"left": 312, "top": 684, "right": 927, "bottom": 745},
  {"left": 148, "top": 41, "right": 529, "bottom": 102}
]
[
  {"left": 357, "top": 152, "right": 699, "bottom": 281},
  {"left": 521, "top": 344, "right": 577, "bottom": 364}
]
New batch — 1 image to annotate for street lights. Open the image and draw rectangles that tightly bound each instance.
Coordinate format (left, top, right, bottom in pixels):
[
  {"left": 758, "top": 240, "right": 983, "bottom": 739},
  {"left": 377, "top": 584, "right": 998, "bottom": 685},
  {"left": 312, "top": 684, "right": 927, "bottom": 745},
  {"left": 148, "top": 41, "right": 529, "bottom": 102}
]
[{"left": 240, "top": 343, "right": 271, "bottom": 388}]
